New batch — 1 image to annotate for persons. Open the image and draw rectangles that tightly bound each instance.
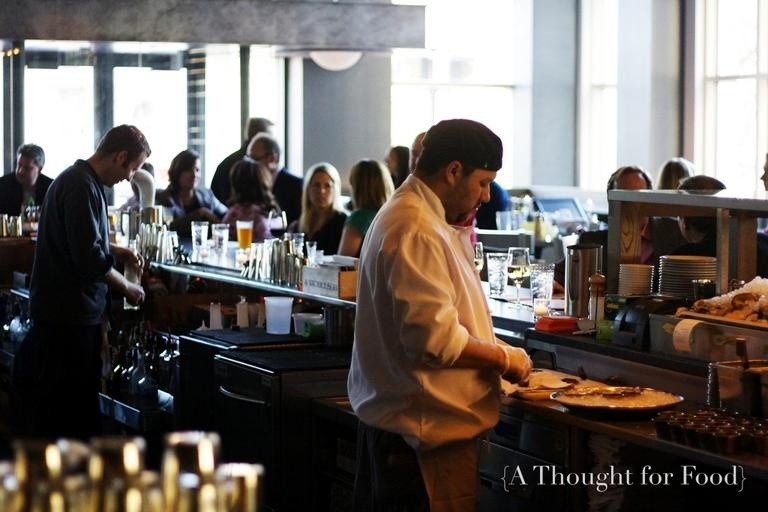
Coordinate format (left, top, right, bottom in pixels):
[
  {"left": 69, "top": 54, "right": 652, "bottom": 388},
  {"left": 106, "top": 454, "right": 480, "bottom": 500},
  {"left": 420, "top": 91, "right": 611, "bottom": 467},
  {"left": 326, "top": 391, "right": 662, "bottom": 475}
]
[
  {"left": 120, "top": 118, "right": 510, "bottom": 263},
  {"left": 2, "top": 145, "right": 56, "bottom": 217},
  {"left": 30, "top": 123, "right": 152, "bottom": 442},
  {"left": 347, "top": 120, "right": 533, "bottom": 512},
  {"left": 554, "top": 153, "right": 767, "bottom": 294}
]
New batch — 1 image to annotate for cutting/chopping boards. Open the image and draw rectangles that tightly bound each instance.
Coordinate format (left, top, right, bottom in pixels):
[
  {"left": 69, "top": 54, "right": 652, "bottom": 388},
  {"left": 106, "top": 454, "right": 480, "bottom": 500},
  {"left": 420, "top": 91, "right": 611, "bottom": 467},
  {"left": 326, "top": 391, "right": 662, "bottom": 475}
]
[{"left": 496, "top": 367, "right": 608, "bottom": 402}]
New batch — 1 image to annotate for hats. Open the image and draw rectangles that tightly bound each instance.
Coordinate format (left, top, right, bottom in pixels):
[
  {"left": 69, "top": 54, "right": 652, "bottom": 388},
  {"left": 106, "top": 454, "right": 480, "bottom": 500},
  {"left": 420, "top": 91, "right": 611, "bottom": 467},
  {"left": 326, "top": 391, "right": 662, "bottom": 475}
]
[{"left": 422, "top": 118, "right": 503, "bottom": 172}]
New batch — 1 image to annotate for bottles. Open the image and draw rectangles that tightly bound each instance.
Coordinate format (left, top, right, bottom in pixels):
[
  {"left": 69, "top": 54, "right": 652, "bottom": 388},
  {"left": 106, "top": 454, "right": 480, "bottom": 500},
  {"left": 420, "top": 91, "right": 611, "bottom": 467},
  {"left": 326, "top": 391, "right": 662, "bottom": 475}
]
[
  {"left": 0, "top": 430, "right": 267, "bottom": 512},
  {"left": 102, "top": 318, "right": 180, "bottom": 410}
]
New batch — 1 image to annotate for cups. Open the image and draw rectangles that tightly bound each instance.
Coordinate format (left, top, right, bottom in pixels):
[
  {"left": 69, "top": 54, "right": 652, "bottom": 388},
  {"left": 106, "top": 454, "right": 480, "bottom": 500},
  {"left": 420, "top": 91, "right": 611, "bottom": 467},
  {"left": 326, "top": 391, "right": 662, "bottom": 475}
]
[
  {"left": 262, "top": 296, "right": 295, "bottom": 335},
  {"left": 268, "top": 210, "right": 288, "bottom": 238},
  {"left": 647, "top": 404, "right": 768, "bottom": 456},
  {"left": 567, "top": 245, "right": 605, "bottom": 318},
  {"left": 473, "top": 241, "right": 556, "bottom": 320},
  {"left": 190, "top": 218, "right": 255, "bottom": 256}
]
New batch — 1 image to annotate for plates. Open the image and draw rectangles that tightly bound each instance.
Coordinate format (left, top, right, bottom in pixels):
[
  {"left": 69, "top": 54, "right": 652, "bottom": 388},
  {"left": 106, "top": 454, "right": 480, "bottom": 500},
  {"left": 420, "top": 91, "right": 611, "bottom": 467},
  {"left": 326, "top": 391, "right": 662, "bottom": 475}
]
[
  {"left": 618, "top": 254, "right": 717, "bottom": 304},
  {"left": 548, "top": 382, "right": 687, "bottom": 411}
]
